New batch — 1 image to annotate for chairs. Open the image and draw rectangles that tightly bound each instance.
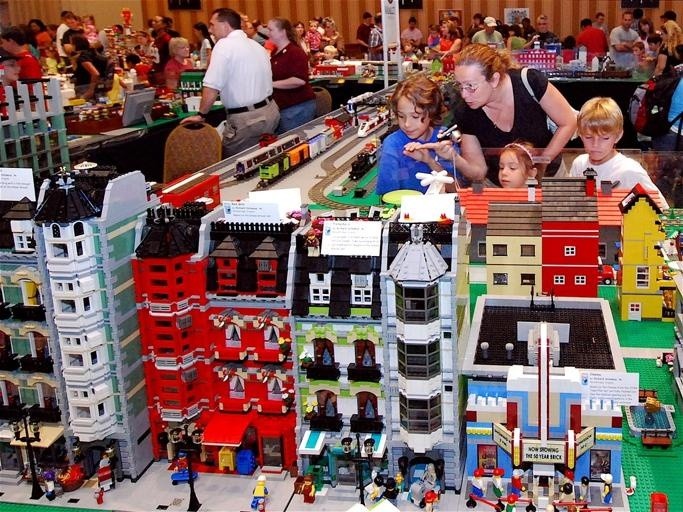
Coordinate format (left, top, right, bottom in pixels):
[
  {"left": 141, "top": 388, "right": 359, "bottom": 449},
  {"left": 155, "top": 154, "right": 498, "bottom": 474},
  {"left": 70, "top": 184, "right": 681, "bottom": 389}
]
[
  {"left": 308, "top": 84, "right": 332, "bottom": 120},
  {"left": 160, "top": 120, "right": 224, "bottom": 188}
]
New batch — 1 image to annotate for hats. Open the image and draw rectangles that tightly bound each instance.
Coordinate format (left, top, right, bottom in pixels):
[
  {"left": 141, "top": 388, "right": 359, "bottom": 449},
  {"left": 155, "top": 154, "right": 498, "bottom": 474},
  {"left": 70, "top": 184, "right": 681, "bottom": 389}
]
[{"left": 484, "top": 16, "right": 498, "bottom": 28}]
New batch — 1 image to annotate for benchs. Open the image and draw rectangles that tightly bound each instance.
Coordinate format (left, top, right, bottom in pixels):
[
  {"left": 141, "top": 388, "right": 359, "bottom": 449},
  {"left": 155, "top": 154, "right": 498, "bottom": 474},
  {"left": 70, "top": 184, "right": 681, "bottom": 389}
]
[
  {"left": 638, "top": 386, "right": 659, "bottom": 402},
  {"left": 637, "top": 427, "right": 674, "bottom": 452}
]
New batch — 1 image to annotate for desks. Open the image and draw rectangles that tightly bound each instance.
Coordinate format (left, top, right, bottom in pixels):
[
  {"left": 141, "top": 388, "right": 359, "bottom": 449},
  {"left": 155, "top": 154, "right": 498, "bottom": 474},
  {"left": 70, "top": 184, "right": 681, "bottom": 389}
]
[
  {"left": 66, "top": 78, "right": 333, "bottom": 186},
  {"left": 308, "top": 72, "right": 653, "bottom": 152}
]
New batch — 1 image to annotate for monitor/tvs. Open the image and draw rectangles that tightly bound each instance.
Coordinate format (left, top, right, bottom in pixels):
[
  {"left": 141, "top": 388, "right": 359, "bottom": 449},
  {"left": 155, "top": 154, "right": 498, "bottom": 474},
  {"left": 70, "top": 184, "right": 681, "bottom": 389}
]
[
  {"left": 620, "top": 0, "right": 660, "bottom": 9},
  {"left": 168, "top": 0, "right": 201, "bottom": 10},
  {"left": 399, "top": 0, "right": 423, "bottom": 9},
  {"left": 122, "top": 88, "right": 156, "bottom": 127}
]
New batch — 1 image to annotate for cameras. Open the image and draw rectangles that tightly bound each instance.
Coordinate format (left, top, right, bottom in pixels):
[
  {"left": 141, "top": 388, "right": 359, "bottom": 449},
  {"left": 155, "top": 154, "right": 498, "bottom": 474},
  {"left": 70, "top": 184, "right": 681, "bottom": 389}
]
[{"left": 436, "top": 123, "right": 461, "bottom": 144}]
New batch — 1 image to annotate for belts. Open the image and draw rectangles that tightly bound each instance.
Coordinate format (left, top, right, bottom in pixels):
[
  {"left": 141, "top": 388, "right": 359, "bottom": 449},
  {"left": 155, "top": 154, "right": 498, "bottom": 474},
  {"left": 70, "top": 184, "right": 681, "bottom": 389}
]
[{"left": 224, "top": 93, "right": 273, "bottom": 115}]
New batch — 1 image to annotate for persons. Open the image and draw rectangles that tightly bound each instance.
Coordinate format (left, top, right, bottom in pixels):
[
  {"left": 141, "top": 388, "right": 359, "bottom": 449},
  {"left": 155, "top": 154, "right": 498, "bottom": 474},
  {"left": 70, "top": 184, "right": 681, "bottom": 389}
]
[
  {"left": 3, "top": 7, "right": 344, "bottom": 158},
  {"left": 558, "top": 473, "right": 613, "bottom": 509},
  {"left": 367, "top": 463, "right": 441, "bottom": 511},
  {"left": 174, "top": 452, "right": 189, "bottom": 473},
  {"left": 251, "top": 475, "right": 269, "bottom": 511},
  {"left": 662, "top": 265, "right": 673, "bottom": 279},
  {"left": 657, "top": 356, "right": 663, "bottom": 367},
  {"left": 377, "top": 42, "right": 669, "bottom": 218},
  {"left": 300, "top": 476, "right": 315, "bottom": 504},
  {"left": 357, "top": 10, "right": 682, "bottom": 151},
  {"left": 471, "top": 467, "right": 525, "bottom": 512}
]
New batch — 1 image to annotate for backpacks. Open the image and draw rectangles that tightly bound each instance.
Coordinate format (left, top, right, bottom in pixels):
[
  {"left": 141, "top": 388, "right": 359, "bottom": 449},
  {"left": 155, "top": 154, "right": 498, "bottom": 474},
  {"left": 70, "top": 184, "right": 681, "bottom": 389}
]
[
  {"left": 626, "top": 70, "right": 683, "bottom": 139},
  {"left": 521, "top": 66, "right": 581, "bottom": 141},
  {"left": 91, "top": 54, "right": 115, "bottom": 94}
]
